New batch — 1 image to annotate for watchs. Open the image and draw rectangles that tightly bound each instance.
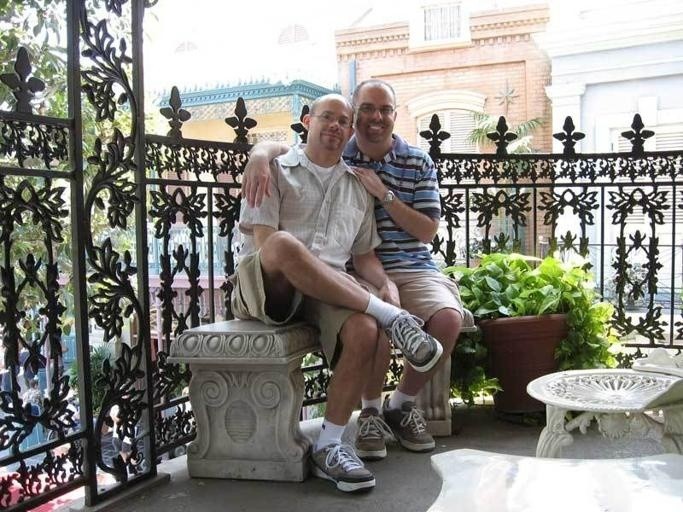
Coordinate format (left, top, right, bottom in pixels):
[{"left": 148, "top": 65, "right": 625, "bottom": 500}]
[{"left": 380, "top": 190, "right": 397, "bottom": 207}]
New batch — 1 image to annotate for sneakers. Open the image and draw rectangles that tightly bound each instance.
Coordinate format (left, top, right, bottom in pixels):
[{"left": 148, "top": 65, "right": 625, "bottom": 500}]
[
  {"left": 311, "top": 398, "right": 436, "bottom": 492},
  {"left": 386, "top": 309, "right": 443, "bottom": 372}
]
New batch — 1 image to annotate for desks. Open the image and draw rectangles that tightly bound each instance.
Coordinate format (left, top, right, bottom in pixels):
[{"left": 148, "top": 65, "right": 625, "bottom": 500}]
[{"left": 523, "top": 364, "right": 682, "bottom": 458}]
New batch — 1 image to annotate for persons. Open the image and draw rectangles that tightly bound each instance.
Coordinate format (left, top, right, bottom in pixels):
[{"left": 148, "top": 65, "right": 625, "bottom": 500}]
[
  {"left": 224, "top": 94, "right": 445, "bottom": 491},
  {"left": 238, "top": 80, "right": 465, "bottom": 460}
]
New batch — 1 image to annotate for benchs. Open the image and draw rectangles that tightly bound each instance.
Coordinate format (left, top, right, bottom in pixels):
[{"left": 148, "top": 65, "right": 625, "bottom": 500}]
[{"left": 165, "top": 306, "right": 479, "bottom": 485}]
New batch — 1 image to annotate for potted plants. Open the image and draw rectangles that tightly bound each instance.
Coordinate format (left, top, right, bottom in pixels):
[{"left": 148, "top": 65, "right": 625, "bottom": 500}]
[{"left": 443, "top": 250, "right": 617, "bottom": 419}]
